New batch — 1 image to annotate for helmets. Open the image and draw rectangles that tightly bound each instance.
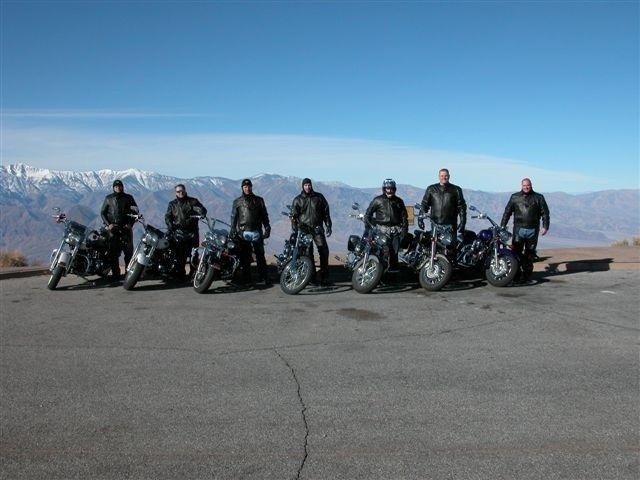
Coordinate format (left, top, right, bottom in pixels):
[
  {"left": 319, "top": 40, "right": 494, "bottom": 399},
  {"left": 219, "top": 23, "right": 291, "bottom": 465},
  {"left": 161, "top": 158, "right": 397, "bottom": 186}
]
[
  {"left": 381, "top": 179, "right": 396, "bottom": 198},
  {"left": 478, "top": 229, "right": 493, "bottom": 244}
]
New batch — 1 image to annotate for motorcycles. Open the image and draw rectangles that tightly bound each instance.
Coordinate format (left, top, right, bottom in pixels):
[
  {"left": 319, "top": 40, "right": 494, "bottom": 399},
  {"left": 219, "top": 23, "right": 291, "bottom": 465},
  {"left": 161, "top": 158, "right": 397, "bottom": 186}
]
[
  {"left": 189, "top": 205, "right": 260, "bottom": 294},
  {"left": 333, "top": 200, "right": 407, "bottom": 295},
  {"left": 273, "top": 205, "right": 327, "bottom": 296},
  {"left": 119, "top": 204, "right": 187, "bottom": 290},
  {"left": 400, "top": 200, "right": 466, "bottom": 291},
  {"left": 45, "top": 205, "right": 112, "bottom": 290},
  {"left": 456, "top": 203, "right": 521, "bottom": 289}
]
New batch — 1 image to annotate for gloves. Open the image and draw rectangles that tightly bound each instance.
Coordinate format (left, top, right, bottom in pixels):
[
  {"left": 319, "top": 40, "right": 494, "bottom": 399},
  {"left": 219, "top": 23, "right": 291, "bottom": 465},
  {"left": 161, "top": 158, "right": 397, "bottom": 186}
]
[
  {"left": 265, "top": 228, "right": 270, "bottom": 238},
  {"left": 417, "top": 219, "right": 425, "bottom": 230},
  {"left": 457, "top": 223, "right": 465, "bottom": 233},
  {"left": 326, "top": 228, "right": 332, "bottom": 237}
]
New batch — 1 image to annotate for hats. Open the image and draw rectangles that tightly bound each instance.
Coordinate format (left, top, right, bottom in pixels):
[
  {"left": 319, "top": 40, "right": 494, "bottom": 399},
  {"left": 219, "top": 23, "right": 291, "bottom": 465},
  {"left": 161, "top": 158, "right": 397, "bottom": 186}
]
[
  {"left": 302, "top": 178, "right": 312, "bottom": 188},
  {"left": 113, "top": 180, "right": 122, "bottom": 187},
  {"left": 241, "top": 179, "right": 252, "bottom": 187}
]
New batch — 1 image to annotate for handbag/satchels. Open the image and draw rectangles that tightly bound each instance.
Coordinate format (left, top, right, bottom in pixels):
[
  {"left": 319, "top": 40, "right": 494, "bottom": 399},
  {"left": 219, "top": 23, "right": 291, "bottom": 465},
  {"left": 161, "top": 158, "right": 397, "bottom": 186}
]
[{"left": 241, "top": 230, "right": 261, "bottom": 242}]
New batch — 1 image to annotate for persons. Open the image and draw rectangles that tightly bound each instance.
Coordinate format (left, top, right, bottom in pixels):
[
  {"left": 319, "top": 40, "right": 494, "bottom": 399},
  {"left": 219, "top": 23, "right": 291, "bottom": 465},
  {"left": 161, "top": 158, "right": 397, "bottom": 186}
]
[
  {"left": 100, "top": 179, "right": 138, "bottom": 282},
  {"left": 165, "top": 184, "right": 207, "bottom": 284},
  {"left": 290, "top": 178, "right": 333, "bottom": 287},
  {"left": 500, "top": 178, "right": 550, "bottom": 281},
  {"left": 231, "top": 179, "right": 271, "bottom": 286},
  {"left": 365, "top": 179, "right": 408, "bottom": 280},
  {"left": 418, "top": 169, "right": 467, "bottom": 284}
]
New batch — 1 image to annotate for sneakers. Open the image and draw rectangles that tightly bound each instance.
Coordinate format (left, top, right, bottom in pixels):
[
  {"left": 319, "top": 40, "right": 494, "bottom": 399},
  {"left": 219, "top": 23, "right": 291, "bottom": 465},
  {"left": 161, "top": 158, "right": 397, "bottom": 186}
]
[
  {"left": 507, "top": 279, "right": 513, "bottom": 286},
  {"left": 527, "top": 280, "right": 538, "bottom": 284}
]
